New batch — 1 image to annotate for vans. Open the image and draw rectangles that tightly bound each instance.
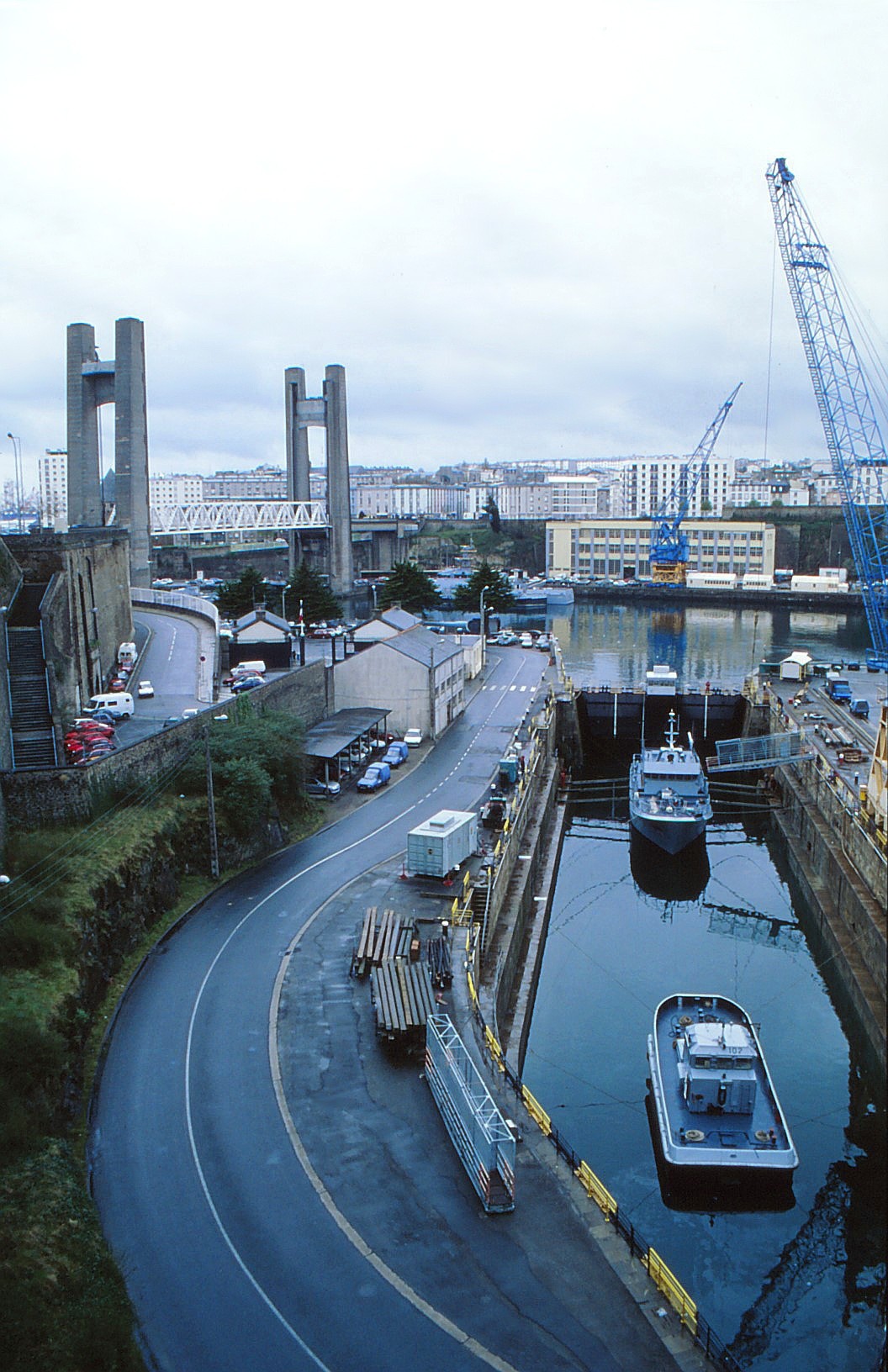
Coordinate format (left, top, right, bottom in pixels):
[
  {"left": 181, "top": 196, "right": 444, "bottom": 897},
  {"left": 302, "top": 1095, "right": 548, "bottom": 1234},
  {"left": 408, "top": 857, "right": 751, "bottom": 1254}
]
[{"left": 83, "top": 693, "right": 134, "bottom": 721}]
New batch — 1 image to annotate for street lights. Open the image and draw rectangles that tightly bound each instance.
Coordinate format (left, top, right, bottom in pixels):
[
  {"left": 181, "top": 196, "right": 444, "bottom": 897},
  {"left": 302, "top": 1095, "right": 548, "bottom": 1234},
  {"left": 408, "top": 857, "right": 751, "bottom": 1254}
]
[
  {"left": 282, "top": 584, "right": 292, "bottom": 620},
  {"left": 371, "top": 584, "right": 379, "bottom": 608},
  {"left": 480, "top": 585, "right": 489, "bottom": 633},
  {"left": 6, "top": 431, "right": 23, "bottom": 534}
]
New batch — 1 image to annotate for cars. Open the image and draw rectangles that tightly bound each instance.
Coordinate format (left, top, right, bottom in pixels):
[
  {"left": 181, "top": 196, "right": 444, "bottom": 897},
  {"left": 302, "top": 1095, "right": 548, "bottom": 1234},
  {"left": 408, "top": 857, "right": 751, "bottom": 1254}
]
[
  {"left": 137, "top": 681, "right": 155, "bottom": 699},
  {"left": 112, "top": 670, "right": 130, "bottom": 683},
  {"left": 163, "top": 715, "right": 183, "bottom": 726},
  {"left": 222, "top": 670, "right": 267, "bottom": 694},
  {"left": 497, "top": 568, "right": 643, "bottom": 587},
  {"left": 63, "top": 708, "right": 122, "bottom": 765},
  {"left": 151, "top": 576, "right": 288, "bottom": 591},
  {"left": 180, "top": 708, "right": 199, "bottom": 718},
  {"left": 108, "top": 679, "right": 127, "bottom": 693},
  {"left": 403, "top": 728, "right": 424, "bottom": 748},
  {"left": 119, "top": 659, "right": 136, "bottom": 673},
  {"left": 849, "top": 699, "right": 869, "bottom": 716},
  {"left": 322, "top": 728, "right": 394, "bottom": 780},
  {"left": 284, "top": 618, "right": 551, "bottom": 652},
  {"left": 303, "top": 776, "right": 340, "bottom": 796}
]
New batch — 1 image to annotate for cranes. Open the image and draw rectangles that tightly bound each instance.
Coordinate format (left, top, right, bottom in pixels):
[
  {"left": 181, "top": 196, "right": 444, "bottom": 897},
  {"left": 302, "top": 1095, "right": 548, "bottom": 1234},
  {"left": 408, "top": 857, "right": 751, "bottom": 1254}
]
[
  {"left": 764, "top": 155, "right": 887, "bottom": 681},
  {"left": 646, "top": 382, "right": 743, "bottom": 589}
]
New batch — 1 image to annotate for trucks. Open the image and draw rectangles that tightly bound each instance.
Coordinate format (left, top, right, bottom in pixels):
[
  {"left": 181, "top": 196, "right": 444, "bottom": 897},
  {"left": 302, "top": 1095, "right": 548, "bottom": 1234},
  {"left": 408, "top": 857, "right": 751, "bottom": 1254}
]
[
  {"left": 117, "top": 642, "right": 138, "bottom": 665},
  {"left": 356, "top": 761, "right": 391, "bottom": 793},
  {"left": 826, "top": 676, "right": 853, "bottom": 705},
  {"left": 381, "top": 741, "right": 409, "bottom": 769},
  {"left": 230, "top": 660, "right": 266, "bottom": 676}
]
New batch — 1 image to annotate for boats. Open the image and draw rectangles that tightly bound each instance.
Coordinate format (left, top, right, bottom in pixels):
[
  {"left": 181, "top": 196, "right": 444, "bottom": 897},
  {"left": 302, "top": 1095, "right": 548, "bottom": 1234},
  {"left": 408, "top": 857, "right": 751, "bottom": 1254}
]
[
  {"left": 646, "top": 993, "right": 801, "bottom": 1170},
  {"left": 628, "top": 707, "right": 714, "bottom": 856}
]
